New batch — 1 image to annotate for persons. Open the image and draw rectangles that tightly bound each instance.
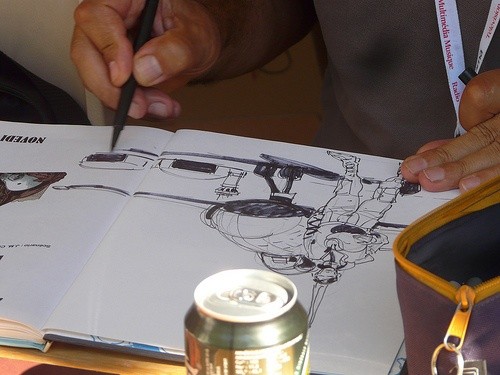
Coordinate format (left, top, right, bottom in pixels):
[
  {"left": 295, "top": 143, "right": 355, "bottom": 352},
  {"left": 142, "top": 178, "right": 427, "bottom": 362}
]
[{"left": 68, "top": 0, "right": 500, "bottom": 196}]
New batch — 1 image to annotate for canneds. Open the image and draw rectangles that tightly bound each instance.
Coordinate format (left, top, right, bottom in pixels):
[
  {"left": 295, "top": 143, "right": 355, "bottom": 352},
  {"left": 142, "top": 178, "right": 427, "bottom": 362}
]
[{"left": 183, "top": 269, "right": 311, "bottom": 374}]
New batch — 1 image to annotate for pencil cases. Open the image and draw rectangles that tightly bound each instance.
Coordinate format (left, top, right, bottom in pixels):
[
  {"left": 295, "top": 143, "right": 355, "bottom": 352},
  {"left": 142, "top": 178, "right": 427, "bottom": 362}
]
[{"left": 392, "top": 175, "right": 499, "bottom": 374}]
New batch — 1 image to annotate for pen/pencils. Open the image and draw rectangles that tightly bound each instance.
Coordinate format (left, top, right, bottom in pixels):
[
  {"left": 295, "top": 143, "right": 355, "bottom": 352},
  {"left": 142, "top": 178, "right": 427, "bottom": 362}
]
[{"left": 109, "top": 0, "right": 160, "bottom": 151}]
